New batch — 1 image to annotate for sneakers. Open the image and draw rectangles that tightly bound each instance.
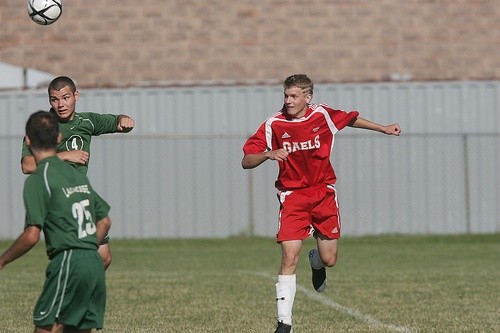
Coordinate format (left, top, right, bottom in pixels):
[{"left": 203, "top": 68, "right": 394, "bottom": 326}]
[
  {"left": 309, "top": 249, "right": 327, "bottom": 293},
  {"left": 274, "top": 317, "right": 293, "bottom": 333}
]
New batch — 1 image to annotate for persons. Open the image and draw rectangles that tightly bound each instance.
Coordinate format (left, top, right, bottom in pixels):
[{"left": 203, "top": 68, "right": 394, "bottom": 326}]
[
  {"left": 241, "top": 74, "right": 401, "bottom": 333},
  {"left": 21, "top": 76, "right": 135, "bottom": 271},
  {"left": 0, "top": 110, "right": 112, "bottom": 333}
]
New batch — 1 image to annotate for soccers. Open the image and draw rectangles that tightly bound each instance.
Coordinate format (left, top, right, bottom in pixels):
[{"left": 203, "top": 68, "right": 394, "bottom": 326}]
[{"left": 27, "top": 0, "right": 63, "bottom": 26}]
